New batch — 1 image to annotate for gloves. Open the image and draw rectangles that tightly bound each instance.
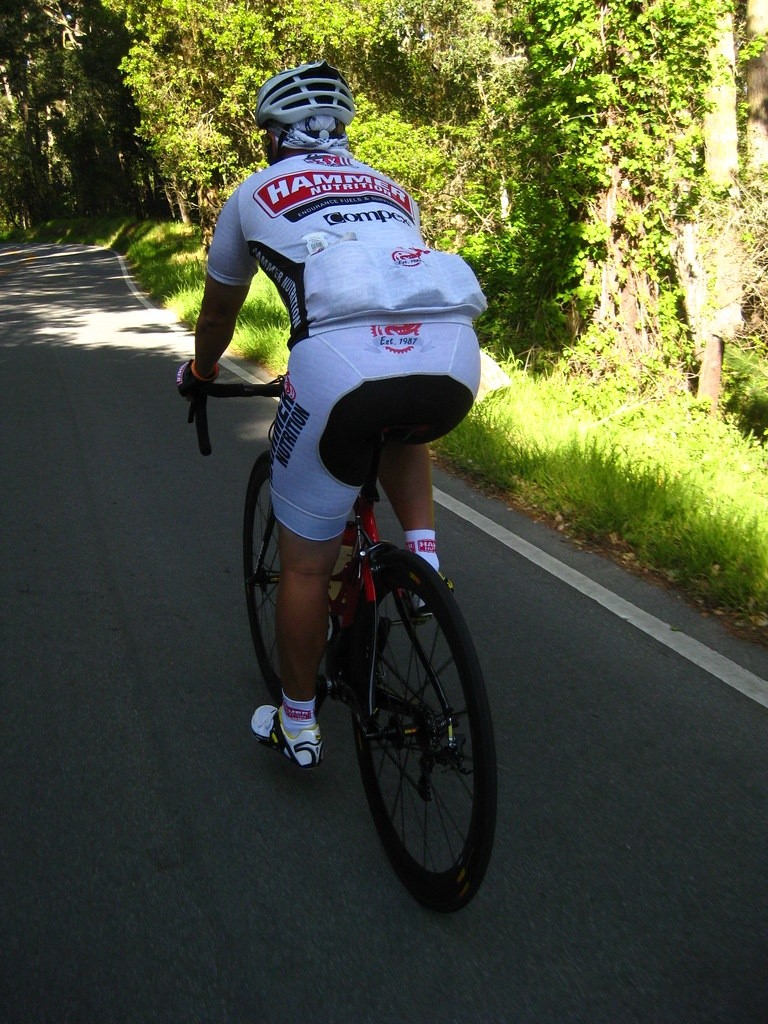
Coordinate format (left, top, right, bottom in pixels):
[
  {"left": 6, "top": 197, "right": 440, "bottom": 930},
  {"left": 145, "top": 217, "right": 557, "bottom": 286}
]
[{"left": 176, "top": 358, "right": 220, "bottom": 396}]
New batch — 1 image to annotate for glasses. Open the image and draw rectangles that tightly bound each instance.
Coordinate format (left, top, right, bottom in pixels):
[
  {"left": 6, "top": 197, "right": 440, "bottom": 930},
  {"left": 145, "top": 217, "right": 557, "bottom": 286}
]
[{"left": 260, "top": 130, "right": 277, "bottom": 157}]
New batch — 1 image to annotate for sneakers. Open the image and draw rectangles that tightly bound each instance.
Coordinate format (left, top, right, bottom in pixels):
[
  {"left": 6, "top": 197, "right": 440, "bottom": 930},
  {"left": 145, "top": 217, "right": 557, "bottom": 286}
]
[
  {"left": 248, "top": 704, "right": 324, "bottom": 768},
  {"left": 397, "top": 573, "right": 454, "bottom": 616}
]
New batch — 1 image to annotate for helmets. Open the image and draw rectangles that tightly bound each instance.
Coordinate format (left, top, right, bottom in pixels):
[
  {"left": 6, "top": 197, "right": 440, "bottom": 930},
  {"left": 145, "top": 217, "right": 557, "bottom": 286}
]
[{"left": 256, "top": 59, "right": 354, "bottom": 129}]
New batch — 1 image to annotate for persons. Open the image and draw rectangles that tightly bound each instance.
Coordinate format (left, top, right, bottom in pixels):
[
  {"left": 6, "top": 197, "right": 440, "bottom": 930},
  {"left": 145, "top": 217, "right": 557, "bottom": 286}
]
[{"left": 178, "top": 60, "right": 481, "bottom": 771}]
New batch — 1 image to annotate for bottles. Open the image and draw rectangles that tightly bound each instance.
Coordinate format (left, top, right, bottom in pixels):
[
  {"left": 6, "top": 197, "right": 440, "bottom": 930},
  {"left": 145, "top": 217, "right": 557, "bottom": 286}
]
[{"left": 328, "top": 521, "right": 355, "bottom": 601}]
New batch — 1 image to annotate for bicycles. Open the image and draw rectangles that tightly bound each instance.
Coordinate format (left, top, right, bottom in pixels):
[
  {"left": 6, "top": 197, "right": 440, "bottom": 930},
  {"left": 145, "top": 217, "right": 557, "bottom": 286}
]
[{"left": 185, "top": 374, "right": 501, "bottom": 915}]
